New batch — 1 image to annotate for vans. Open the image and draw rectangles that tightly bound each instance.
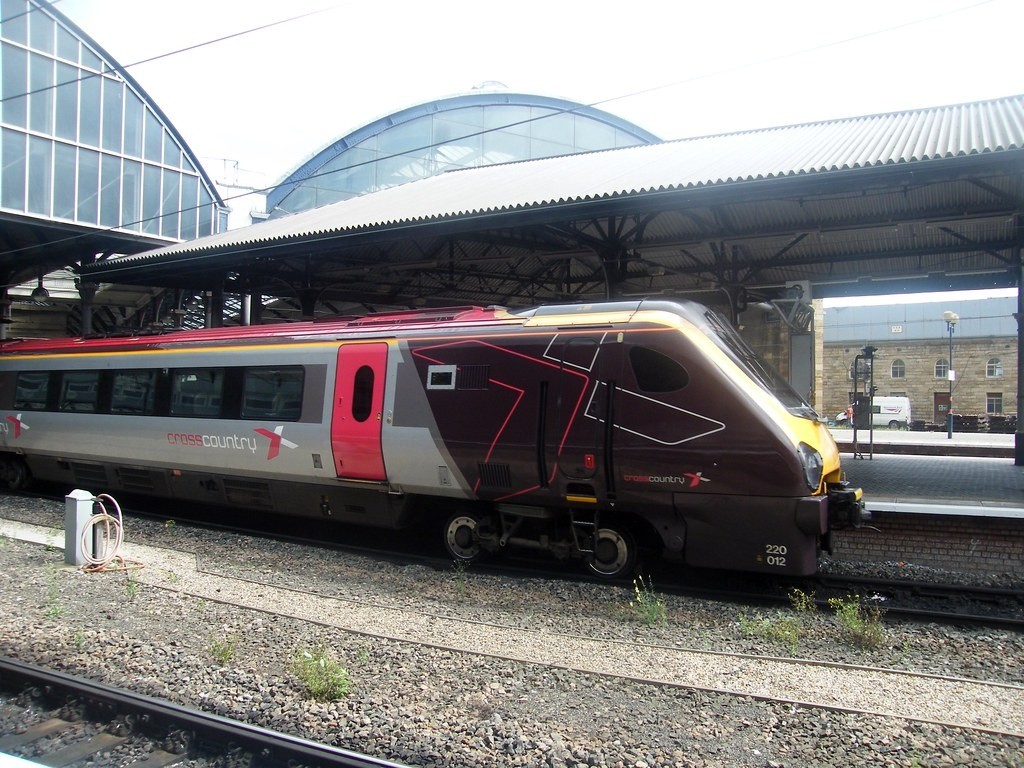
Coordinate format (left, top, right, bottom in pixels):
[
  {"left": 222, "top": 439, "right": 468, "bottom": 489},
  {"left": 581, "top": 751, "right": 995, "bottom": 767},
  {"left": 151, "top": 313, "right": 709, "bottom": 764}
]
[{"left": 834, "top": 394, "right": 911, "bottom": 429}]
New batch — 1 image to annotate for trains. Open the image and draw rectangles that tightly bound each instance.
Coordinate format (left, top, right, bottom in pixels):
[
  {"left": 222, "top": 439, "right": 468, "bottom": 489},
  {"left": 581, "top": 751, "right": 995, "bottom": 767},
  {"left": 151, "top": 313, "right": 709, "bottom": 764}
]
[{"left": 1, "top": 297, "right": 882, "bottom": 588}]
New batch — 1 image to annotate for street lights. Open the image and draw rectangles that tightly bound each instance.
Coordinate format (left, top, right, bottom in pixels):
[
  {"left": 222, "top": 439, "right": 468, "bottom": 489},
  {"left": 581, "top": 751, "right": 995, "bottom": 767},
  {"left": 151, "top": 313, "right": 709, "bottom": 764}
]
[{"left": 943, "top": 311, "right": 958, "bottom": 439}]
